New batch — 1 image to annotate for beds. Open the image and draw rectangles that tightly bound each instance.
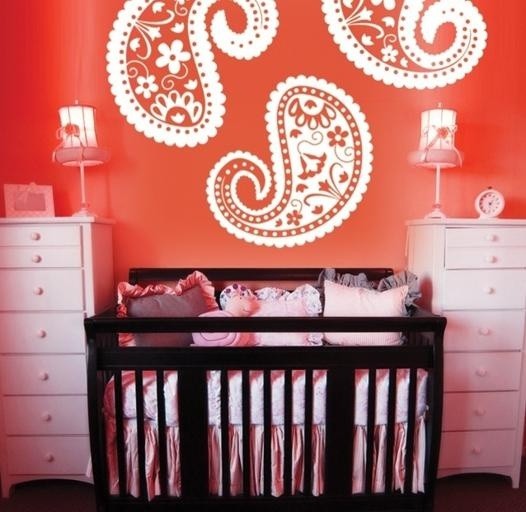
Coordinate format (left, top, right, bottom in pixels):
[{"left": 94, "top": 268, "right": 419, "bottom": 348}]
[{"left": 83, "top": 266, "right": 448, "bottom": 512}]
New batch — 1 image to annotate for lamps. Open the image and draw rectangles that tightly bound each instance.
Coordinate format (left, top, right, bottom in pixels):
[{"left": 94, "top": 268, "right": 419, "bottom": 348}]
[
  {"left": 407, "top": 100, "right": 466, "bottom": 220},
  {"left": 50, "top": 98, "right": 109, "bottom": 218}
]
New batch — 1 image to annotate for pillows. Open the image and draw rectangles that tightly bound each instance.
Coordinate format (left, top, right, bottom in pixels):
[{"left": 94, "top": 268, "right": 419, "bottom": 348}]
[
  {"left": 313, "top": 267, "right": 423, "bottom": 347},
  {"left": 114, "top": 269, "right": 218, "bottom": 348},
  {"left": 247, "top": 280, "right": 324, "bottom": 346}
]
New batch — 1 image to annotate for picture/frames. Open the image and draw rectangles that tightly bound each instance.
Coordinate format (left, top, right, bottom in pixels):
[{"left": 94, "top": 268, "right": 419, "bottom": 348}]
[{"left": 2, "top": 182, "right": 55, "bottom": 218}]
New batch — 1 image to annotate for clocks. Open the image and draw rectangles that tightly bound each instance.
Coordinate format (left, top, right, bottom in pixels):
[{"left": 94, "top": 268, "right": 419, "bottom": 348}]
[{"left": 473, "top": 186, "right": 505, "bottom": 219}]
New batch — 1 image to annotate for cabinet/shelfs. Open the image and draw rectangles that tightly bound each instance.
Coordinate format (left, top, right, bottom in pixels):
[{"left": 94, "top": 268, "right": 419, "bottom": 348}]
[
  {"left": 0, "top": 214, "right": 117, "bottom": 500},
  {"left": 404, "top": 218, "right": 525, "bottom": 491}
]
